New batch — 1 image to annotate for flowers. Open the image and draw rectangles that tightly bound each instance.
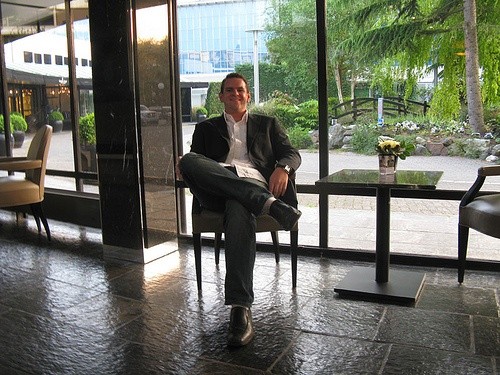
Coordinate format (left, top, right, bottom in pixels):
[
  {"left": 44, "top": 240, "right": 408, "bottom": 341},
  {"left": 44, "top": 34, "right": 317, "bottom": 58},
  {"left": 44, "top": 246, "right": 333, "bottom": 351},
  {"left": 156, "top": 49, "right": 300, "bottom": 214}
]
[{"left": 377, "top": 138, "right": 416, "bottom": 161}]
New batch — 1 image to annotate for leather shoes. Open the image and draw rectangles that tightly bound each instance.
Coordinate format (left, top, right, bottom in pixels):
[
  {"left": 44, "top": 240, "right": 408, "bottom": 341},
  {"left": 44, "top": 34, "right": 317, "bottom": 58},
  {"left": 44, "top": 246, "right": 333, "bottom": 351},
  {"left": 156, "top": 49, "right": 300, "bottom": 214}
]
[
  {"left": 268, "top": 199, "right": 302, "bottom": 231},
  {"left": 225, "top": 306, "right": 254, "bottom": 346}
]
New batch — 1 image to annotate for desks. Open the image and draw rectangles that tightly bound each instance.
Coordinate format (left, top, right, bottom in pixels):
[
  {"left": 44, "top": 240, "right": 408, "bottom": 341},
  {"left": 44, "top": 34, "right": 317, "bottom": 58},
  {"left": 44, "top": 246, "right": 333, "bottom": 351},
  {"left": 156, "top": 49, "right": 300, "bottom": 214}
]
[{"left": 315, "top": 168, "right": 444, "bottom": 308}]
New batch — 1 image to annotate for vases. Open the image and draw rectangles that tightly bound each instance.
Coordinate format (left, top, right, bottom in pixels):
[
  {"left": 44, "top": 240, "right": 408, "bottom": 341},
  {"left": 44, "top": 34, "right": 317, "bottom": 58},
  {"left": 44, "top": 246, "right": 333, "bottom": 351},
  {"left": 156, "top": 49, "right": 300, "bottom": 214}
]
[{"left": 378, "top": 154, "right": 397, "bottom": 174}]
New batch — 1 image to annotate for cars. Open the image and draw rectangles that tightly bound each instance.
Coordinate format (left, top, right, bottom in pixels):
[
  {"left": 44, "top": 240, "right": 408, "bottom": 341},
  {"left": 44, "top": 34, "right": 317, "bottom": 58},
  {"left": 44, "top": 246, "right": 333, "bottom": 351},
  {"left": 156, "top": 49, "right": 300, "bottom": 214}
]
[{"left": 140, "top": 105, "right": 159, "bottom": 124}]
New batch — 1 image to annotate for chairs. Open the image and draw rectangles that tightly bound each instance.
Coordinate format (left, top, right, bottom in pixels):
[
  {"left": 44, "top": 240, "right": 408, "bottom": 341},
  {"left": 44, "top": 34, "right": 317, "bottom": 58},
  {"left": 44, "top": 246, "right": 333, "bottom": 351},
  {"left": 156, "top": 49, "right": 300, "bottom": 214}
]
[
  {"left": 0, "top": 125, "right": 52, "bottom": 241},
  {"left": 192, "top": 162, "right": 299, "bottom": 300},
  {"left": 457, "top": 166, "right": 500, "bottom": 285}
]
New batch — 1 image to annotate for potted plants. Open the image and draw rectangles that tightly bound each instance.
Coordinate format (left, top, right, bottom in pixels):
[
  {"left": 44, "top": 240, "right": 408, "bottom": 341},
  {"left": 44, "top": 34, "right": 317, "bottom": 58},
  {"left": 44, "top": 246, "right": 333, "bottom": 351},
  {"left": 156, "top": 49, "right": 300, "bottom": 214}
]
[
  {"left": 0, "top": 114, "right": 15, "bottom": 156},
  {"left": 196, "top": 108, "right": 208, "bottom": 121},
  {"left": 47, "top": 111, "right": 64, "bottom": 132},
  {"left": 10, "top": 114, "right": 27, "bottom": 148},
  {"left": 79, "top": 112, "right": 100, "bottom": 186}
]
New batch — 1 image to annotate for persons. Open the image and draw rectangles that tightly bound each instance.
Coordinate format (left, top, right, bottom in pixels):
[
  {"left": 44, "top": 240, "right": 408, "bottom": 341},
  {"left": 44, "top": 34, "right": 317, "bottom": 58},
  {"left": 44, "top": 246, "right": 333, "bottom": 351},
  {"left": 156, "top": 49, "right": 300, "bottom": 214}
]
[{"left": 173, "top": 73, "right": 303, "bottom": 349}]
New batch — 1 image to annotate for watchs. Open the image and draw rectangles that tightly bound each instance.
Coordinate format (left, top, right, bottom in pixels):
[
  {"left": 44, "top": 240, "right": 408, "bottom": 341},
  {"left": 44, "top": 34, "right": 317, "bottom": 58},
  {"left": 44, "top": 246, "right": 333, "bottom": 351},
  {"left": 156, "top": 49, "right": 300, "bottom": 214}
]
[{"left": 274, "top": 163, "right": 292, "bottom": 176}]
